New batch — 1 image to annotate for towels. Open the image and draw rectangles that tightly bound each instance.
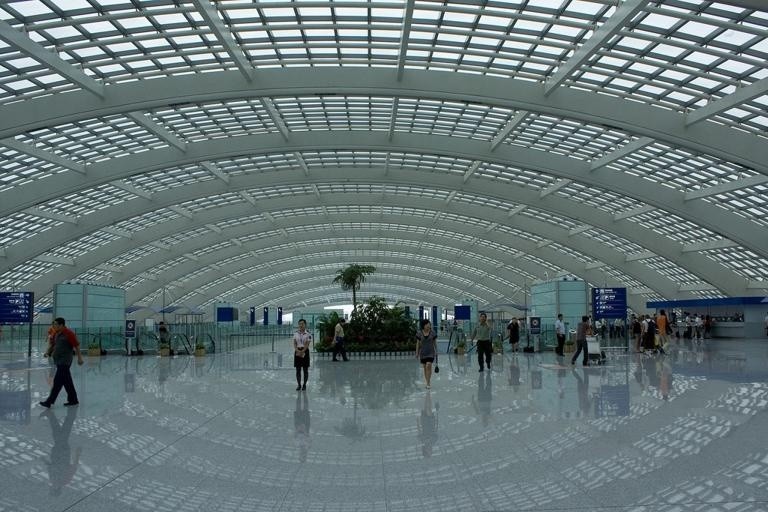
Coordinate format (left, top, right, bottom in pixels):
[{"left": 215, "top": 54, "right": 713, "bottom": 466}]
[{"left": 568, "top": 330, "right": 577, "bottom": 351}]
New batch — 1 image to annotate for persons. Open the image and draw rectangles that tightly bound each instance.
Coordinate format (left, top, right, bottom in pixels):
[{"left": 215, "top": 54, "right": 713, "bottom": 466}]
[
  {"left": 157, "top": 321, "right": 179, "bottom": 358},
  {"left": 571, "top": 316, "right": 591, "bottom": 366},
  {"left": 634, "top": 356, "right": 672, "bottom": 398},
  {"left": 555, "top": 352, "right": 566, "bottom": 400},
  {"left": 416, "top": 320, "right": 439, "bottom": 386},
  {"left": 591, "top": 310, "right": 745, "bottom": 354},
  {"left": 507, "top": 317, "right": 520, "bottom": 353},
  {"left": 295, "top": 396, "right": 311, "bottom": 463},
  {"left": 39, "top": 407, "right": 82, "bottom": 495},
  {"left": 418, "top": 388, "right": 441, "bottom": 458},
  {"left": 40, "top": 318, "right": 82, "bottom": 406},
  {"left": 471, "top": 314, "right": 493, "bottom": 371},
  {"left": 573, "top": 368, "right": 596, "bottom": 424},
  {"left": 294, "top": 319, "right": 310, "bottom": 393},
  {"left": 473, "top": 374, "right": 493, "bottom": 427},
  {"left": 506, "top": 358, "right": 522, "bottom": 393},
  {"left": 333, "top": 319, "right": 349, "bottom": 362},
  {"left": 555, "top": 314, "right": 566, "bottom": 356}
]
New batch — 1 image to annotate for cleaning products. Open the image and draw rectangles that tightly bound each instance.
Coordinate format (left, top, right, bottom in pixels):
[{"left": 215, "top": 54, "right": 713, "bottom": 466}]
[{"left": 586, "top": 334, "right": 606, "bottom": 365}]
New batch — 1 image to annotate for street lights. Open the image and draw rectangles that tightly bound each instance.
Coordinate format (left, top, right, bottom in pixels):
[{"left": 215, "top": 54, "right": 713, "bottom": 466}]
[
  {"left": 435, "top": 367, "right": 439, "bottom": 372},
  {"left": 665, "top": 322, "right": 672, "bottom": 335}
]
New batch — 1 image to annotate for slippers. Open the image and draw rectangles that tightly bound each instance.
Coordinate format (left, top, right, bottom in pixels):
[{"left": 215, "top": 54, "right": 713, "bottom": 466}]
[
  {"left": 633, "top": 322, "right": 641, "bottom": 334},
  {"left": 646, "top": 320, "right": 656, "bottom": 334}
]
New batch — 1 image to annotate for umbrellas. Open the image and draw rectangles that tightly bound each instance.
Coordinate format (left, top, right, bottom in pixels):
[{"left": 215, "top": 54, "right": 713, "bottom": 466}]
[
  {"left": 302, "top": 385, "right": 306, "bottom": 390},
  {"left": 296, "top": 384, "right": 301, "bottom": 390}
]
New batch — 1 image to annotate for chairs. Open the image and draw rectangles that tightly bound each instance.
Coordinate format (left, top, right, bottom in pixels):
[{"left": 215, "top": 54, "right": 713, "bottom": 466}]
[
  {"left": 40, "top": 401, "right": 50, "bottom": 408},
  {"left": 479, "top": 362, "right": 490, "bottom": 371},
  {"left": 64, "top": 402, "right": 76, "bottom": 405}
]
